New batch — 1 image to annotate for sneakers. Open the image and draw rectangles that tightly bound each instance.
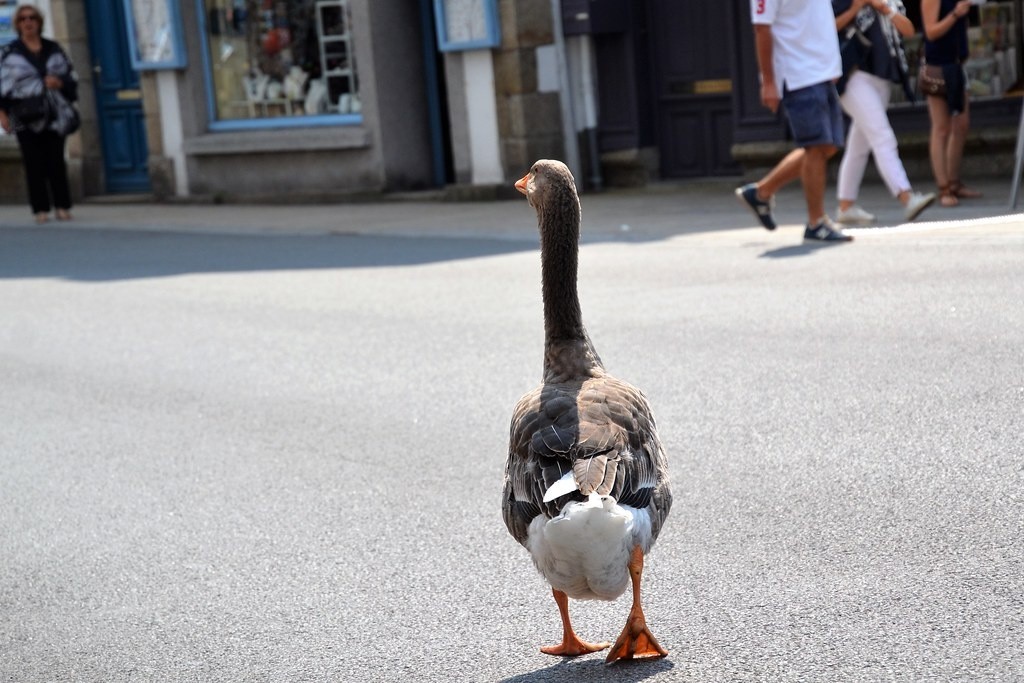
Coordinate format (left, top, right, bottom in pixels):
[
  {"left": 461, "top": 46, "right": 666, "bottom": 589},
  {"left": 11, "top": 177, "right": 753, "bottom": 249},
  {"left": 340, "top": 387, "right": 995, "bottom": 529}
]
[
  {"left": 802, "top": 215, "right": 854, "bottom": 244},
  {"left": 734, "top": 182, "right": 778, "bottom": 230}
]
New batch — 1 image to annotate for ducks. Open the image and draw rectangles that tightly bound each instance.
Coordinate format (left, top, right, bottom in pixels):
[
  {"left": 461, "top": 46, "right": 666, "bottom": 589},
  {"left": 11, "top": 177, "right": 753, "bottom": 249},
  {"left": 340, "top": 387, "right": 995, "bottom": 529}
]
[{"left": 502, "top": 159, "right": 673, "bottom": 667}]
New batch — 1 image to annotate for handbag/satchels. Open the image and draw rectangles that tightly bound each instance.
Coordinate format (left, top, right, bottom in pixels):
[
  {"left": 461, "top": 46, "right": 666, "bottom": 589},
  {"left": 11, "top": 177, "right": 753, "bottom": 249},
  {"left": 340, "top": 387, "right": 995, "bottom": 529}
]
[{"left": 917, "top": 62, "right": 948, "bottom": 96}]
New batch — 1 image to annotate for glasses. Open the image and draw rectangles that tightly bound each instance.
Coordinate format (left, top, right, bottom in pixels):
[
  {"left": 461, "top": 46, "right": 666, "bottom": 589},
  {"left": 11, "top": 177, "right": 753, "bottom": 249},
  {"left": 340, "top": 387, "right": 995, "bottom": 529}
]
[{"left": 16, "top": 12, "right": 40, "bottom": 23}]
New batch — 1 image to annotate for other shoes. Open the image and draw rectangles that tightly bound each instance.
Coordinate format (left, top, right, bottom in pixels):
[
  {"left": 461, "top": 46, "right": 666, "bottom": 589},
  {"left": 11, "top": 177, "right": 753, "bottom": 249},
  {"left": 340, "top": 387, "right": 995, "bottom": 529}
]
[
  {"left": 54, "top": 210, "right": 73, "bottom": 221},
  {"left": 903, "top": 191, "right": 938, "bottom": 222},
  {"left": 938, "top": 185, "right": 958, "bottom": 208},
  {"left": 951, "top": 180, "right": 984, "bottom": 199},
  {"left": 836, "top": 202, "right": 875, "bottom": 223},
  {"left": 35, "top": 212, "right": 50, "bottom": 226}
]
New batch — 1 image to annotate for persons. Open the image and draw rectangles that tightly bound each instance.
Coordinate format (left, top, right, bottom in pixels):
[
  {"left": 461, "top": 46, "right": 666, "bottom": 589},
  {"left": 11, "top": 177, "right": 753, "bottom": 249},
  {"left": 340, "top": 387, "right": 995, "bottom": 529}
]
[
  {"left": 0, "top": 4, "right": 81, "bottom": 225},
  {"left": 735, "top": 0, "right": 984, "bottom": 245}
]
